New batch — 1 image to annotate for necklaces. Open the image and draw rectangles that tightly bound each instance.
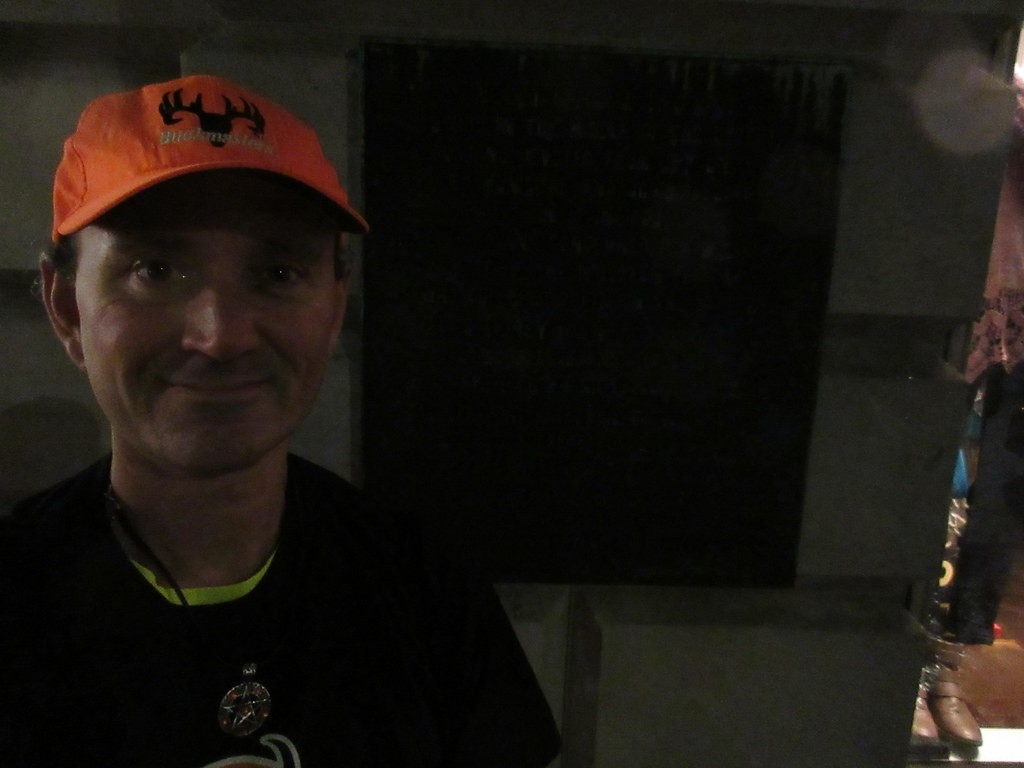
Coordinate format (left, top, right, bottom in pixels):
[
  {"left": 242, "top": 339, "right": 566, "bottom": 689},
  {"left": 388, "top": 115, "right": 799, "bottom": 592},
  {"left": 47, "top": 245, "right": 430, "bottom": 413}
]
[{"left": 94, "top": 470, "right": 294, "bottom": 737}]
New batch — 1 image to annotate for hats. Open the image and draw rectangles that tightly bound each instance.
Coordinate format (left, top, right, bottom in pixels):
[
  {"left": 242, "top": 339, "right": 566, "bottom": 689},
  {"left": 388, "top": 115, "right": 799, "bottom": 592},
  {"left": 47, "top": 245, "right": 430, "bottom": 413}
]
[{"left": 50, "top": 75, "right": 368, "bottom": 246}]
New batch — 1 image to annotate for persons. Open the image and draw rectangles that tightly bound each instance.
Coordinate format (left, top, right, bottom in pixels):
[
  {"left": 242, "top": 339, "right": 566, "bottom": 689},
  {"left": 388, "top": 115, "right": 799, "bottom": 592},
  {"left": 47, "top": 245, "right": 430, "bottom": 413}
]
[
  {"left": 0, "top": 77, "right": 563, "bottom": 768},
  {"left": 905, "top": 29, "right": 1024, "bottom": 748}
]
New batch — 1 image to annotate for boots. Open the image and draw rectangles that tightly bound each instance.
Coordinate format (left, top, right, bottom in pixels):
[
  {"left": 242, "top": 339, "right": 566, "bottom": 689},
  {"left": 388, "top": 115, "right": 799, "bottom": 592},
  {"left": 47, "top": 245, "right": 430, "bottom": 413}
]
[{"left": 910, "top": 636, "right": 982, "bottom": 746}]
